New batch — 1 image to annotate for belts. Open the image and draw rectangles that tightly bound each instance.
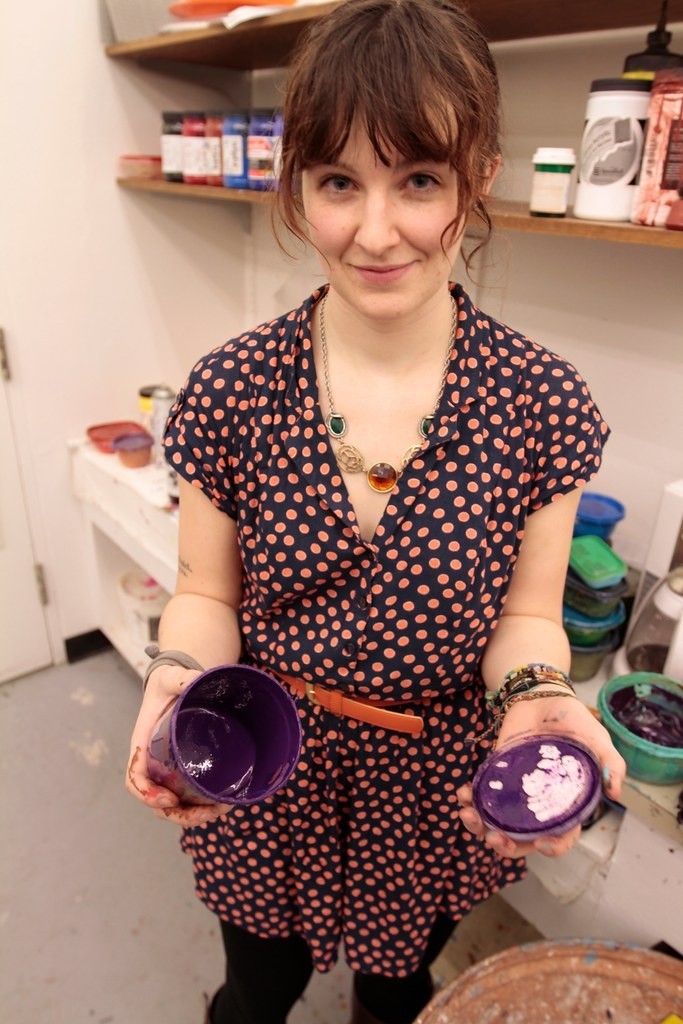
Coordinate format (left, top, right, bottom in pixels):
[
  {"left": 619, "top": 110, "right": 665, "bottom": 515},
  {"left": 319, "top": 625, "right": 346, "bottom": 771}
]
[{"left": 265, "top": 671, "right": 427, "bottom": 734}]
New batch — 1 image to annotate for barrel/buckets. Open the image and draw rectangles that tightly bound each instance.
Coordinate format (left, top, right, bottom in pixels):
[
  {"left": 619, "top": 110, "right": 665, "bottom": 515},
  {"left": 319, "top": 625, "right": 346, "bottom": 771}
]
[{"left": 115, "top": 564, "right": 172, "bottom": 648}]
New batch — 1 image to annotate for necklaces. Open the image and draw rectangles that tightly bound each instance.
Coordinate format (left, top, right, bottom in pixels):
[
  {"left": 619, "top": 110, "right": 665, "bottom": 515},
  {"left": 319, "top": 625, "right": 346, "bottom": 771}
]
[{"left": 319, "top": 293, "right": 457, "bottom": 494}]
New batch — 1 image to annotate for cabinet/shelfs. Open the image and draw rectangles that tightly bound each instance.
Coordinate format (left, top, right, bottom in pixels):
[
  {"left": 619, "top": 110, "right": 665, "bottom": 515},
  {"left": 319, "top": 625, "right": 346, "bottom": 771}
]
[{"left": 67, "top": 0, "right": 681, "bottom": 969}]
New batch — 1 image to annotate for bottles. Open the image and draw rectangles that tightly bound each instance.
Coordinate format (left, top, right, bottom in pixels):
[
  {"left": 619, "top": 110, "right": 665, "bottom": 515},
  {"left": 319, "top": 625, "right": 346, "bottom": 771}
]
[
  {"left": 139, "top": 385, "right": 176, "bottom": 469},
  {"left": 159, "top": 109, "right": 301, "bottom": 193},
  {"left": 573, "top": 0, "right": 683, "bottom": 233}
]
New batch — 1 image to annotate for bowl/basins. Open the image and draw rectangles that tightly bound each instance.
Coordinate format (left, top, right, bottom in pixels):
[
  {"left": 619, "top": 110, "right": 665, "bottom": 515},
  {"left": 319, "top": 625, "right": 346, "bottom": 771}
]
[
  {"left": 110, "top": 433, "right": 152, "bottom": 468},
  {"left": 596, "top": 671, "right": 683, "bottom": 786},
  {"left": 570, "top": 629, "right": 621, "bottom": 683},
  {"left": 562, "top": 600, "right": 625, "bottom": 644},
  {"left": 562, "top": 534, "right": 630, "bottom": 618},
  {"left": 572, "top": 494, "right": 626, "bottom": 541}
]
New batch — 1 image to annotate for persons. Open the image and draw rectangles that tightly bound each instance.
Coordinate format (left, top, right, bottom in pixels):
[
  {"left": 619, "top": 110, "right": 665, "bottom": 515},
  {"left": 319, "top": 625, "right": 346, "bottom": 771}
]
[{"left": 124, "top": 0, "right": 627, "bottom": 1024}]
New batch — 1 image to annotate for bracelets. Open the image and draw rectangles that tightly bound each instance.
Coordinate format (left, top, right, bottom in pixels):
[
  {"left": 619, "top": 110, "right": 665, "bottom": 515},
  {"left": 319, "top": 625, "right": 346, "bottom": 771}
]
[
  {"left": 141, "top": 645, "right": 205, "bottom": 693},
  {"left": 464, "top": 663, "right": 575, "bottom": 752}
]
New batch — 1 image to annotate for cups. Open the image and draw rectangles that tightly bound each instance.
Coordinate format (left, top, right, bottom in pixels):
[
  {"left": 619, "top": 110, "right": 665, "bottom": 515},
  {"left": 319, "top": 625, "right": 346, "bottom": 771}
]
[{"left": 147, "top": 664, "right": 301, "bottom": 805}]
